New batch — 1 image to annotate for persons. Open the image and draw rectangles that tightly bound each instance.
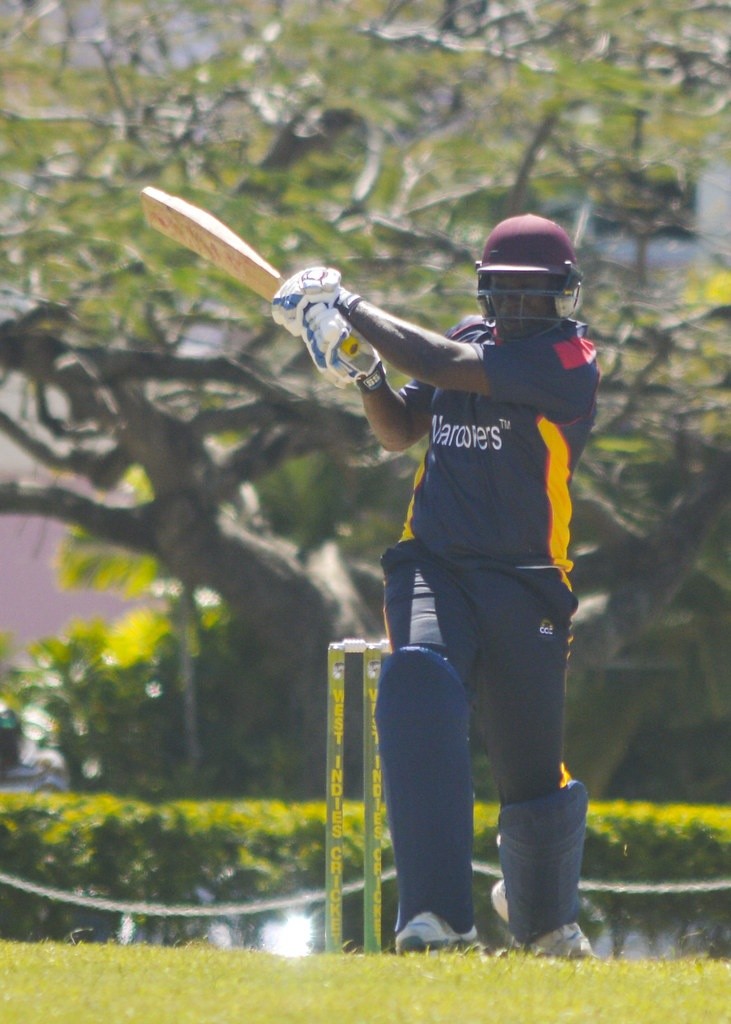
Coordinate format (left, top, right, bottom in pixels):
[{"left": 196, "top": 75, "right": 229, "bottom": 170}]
[
  {"left": 0, "top": 707, "right": 69, "bottom": 793},
  {"left": 272, "top": 214, "right": 601, "bottom": 958}
]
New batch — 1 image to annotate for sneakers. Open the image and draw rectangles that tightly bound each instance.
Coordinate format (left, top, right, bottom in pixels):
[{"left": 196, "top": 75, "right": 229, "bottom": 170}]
[
  {"left": 491, "top": 880, "right": 592, "bottom": 959},
  {"left": 396, "top": 913, "right": 480, "bottom": 952}
]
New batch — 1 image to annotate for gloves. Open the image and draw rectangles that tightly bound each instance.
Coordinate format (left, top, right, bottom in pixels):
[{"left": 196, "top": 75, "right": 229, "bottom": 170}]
[
  {"left": 270, "top": 267, "right": 363, "bottom": 336},
  {"left": 301, "top": 303, "right": 386, "bottom": 392}
]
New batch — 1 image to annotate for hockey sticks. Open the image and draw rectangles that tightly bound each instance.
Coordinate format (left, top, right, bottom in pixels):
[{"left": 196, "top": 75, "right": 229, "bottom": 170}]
[{"left": 141, "top": 185, "right": 375, "bottom": 369}]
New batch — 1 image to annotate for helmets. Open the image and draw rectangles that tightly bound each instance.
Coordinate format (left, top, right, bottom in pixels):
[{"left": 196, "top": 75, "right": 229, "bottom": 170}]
[{"left": 475, "top": 213, "right": 584, "bottom": 343}]
